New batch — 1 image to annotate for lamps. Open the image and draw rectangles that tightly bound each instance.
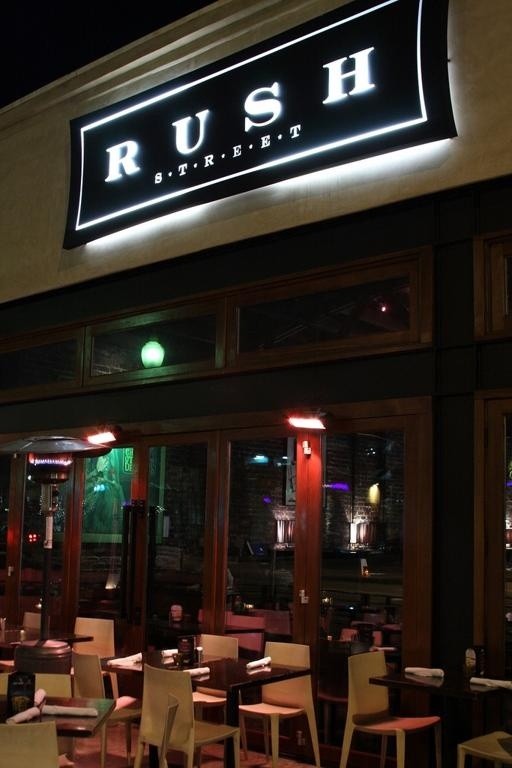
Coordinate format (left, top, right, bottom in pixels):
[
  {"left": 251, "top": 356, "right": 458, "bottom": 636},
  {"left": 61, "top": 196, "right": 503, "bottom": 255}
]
[{"left": 141, "top": 340, "right": 166, "bottom": 369}]
